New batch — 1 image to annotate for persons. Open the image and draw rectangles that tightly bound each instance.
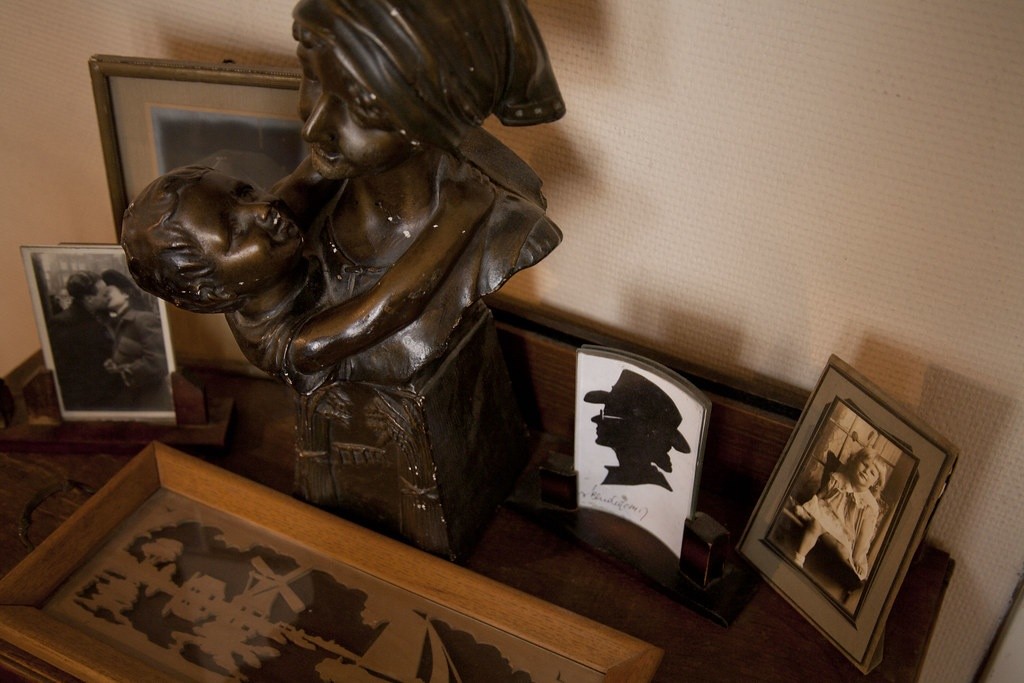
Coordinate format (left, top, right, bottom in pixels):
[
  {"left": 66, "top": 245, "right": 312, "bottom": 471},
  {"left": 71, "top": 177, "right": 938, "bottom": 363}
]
[
  {"left": 788, "top": 448, "right": 886, "bottom": 581},
  {"left": 122, "top": 0, "right": 565, "bottom": 559},
  {"left": 46, "top": 270, "right": 117, "bottom": 412},
  {"left": 100, "top": 269, "right": 174, "bottom": 414}
]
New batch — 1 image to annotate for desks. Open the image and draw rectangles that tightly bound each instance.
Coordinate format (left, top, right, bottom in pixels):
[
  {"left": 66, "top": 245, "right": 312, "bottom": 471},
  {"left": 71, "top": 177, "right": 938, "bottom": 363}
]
[{"left": 0, "top": 319, "right": 957, "bottom": 682}]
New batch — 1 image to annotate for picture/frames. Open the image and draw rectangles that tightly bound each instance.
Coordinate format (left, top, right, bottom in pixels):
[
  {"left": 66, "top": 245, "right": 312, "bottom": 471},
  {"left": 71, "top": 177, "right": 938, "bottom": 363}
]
[
  {"left": 0, "top": 439, "right": 665, "bottom": 683},
  {"left": 734, "top": 353, "right": 959, "bottom": 673},
  {"left": 86, "top": 58, "right": 305, "bottom": 378}
]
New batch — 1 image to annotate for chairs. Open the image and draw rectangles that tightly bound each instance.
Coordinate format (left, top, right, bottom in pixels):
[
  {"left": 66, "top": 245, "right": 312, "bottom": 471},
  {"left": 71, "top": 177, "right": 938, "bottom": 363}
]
[{"left": 790, "top": 450, "right": 889, "bottom": 604}]
